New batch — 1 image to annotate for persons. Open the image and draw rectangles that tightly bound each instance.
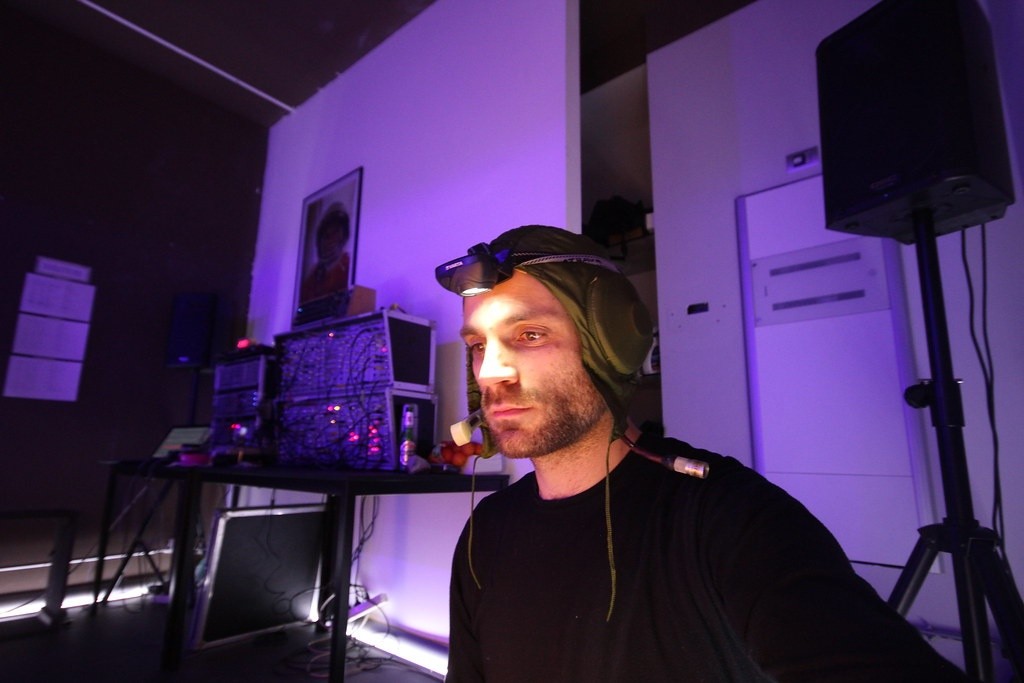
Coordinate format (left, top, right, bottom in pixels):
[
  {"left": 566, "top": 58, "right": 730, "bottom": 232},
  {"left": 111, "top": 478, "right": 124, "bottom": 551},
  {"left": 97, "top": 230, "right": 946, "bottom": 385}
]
[
  {"left": 299, "top": 201, "right": 350, "bottom": 303},
  {"left": 441, "top": 222, "right": 982, "bottom": 683}
]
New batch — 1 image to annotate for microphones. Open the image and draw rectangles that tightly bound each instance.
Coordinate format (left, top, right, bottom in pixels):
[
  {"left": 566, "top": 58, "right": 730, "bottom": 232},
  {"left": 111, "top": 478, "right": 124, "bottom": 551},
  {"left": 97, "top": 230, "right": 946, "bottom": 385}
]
[
  {"left": 449, "top": 408, "right": 485, "bottom": 448},
  {"left": 619, "top": 433, "right": 710, "bottom": 480}
]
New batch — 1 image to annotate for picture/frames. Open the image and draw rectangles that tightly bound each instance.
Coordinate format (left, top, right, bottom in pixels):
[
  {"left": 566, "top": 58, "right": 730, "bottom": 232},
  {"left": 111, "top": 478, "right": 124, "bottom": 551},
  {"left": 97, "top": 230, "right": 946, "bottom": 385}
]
[{"left": 290, "top": 166, "right": 362, "bottom": 331}]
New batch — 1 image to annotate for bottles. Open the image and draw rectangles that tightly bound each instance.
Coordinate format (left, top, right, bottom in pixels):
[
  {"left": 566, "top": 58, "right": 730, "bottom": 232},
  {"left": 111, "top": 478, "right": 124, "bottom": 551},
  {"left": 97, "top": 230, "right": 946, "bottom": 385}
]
[{"left": 398, "top": 406, "right": 417, "bottom": 472}]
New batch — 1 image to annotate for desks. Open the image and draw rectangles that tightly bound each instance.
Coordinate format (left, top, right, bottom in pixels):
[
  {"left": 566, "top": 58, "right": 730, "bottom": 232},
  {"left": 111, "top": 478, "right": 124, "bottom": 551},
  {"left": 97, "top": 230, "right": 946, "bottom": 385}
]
[{"left": 93, "top": 456, "right": 511, "bottom": 680}]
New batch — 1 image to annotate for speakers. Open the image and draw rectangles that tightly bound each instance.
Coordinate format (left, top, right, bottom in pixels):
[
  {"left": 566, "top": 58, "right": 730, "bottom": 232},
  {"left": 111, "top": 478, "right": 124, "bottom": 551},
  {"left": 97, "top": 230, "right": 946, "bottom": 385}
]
[
  {"left": 163, "top": 287, "right": 233, "bottom": 370},
  {"left": 815, "top": 1, "right": 1016, "bottom": 245}
]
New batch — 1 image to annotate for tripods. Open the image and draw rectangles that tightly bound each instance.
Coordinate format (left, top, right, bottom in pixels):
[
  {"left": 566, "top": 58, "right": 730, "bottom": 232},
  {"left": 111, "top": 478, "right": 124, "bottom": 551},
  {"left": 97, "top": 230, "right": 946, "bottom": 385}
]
[
  {"left": 883, "top": 238, "right": 1024, "bottom": 683},
  {"left": 97, "top": 365, "right": 203, "bottom": 609}
]
